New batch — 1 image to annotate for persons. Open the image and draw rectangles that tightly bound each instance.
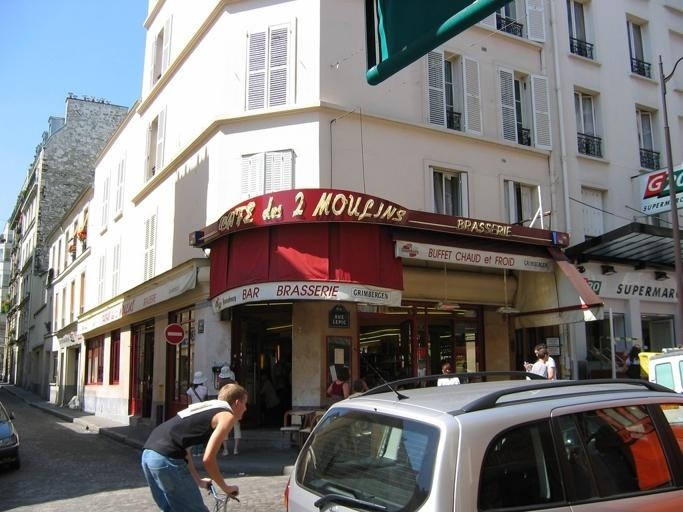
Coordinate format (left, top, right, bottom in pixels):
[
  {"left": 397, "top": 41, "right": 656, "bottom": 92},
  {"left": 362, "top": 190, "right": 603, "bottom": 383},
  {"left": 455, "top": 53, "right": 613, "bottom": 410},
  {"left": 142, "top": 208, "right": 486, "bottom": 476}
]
[
  {"left": 626, "top": 343, "right": 642, "bottom": 379},
  {"left": 346, "top": 380, "right": 368, "bottom": 434},
  {"left": 260, "top": 373, "right": 281, "bottom": 427},
  {"left": 524, "top": 344, "right": 557, "bottom": 380},
  {"left": 141, "top": 384, "right": 248, "bottom": 512},
  {"left": 218, "top": 366, "right": 242, "bottom": 457},
  {"left": 437, "top": 362, "right": 461, "bottom": 386},
  {"left": 186, "top": 371, "right": 209, "bottom": 456},
  {"left": 526, "top": 348, "right": 551, "bottom": 381},
  {"left": 326, "top": 367, "right": 350, "bottom": 410}
]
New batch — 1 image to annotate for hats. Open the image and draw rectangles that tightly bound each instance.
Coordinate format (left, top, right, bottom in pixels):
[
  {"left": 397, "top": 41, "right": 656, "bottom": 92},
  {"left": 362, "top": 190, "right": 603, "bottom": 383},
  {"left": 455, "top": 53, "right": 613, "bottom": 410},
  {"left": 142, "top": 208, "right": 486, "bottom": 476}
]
[
  {"left": 218, "top": 366, "right": 235, "bottom": 378},
  {"left": 192, "top": 371, "right": 208, "bottom": 385}
]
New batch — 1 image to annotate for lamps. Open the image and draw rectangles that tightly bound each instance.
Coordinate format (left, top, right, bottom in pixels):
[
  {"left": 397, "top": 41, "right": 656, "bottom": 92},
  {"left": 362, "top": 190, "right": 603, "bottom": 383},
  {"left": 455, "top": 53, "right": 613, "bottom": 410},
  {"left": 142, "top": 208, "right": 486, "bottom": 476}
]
[
  {"left": 497, "top": 270, "right": 521, "bottom": 314},
  {"left": 601, "top": 265, "right": 616, "bottom": 275},
  {"left": 437, "top": 264, "right": 461, "bottom": 311},
  {"left": 654, "top": 271, "right": 670, "bottom": 281}
]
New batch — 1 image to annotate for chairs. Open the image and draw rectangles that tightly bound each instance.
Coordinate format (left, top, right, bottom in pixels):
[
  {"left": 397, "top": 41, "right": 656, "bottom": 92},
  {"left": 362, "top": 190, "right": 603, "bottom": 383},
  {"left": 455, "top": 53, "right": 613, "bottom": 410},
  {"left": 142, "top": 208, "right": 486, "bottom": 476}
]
[{"left": 281, "top": 411, "right": 324, "bottom": 452}]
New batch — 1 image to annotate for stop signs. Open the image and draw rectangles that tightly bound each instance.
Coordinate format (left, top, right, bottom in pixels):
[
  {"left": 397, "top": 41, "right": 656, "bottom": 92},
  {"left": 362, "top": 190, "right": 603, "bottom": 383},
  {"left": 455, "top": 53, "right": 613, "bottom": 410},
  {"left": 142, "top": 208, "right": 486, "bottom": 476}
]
[{"left": 164, "top": 323, "right": 184, "bottom": 345}]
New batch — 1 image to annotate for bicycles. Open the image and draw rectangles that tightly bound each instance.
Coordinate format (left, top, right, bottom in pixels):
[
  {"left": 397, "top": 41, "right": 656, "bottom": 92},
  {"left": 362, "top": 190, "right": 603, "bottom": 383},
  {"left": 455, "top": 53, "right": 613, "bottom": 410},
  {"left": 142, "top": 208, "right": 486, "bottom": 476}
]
[{"left": 199, "top": 480, "right": 240, "bottom": 512}]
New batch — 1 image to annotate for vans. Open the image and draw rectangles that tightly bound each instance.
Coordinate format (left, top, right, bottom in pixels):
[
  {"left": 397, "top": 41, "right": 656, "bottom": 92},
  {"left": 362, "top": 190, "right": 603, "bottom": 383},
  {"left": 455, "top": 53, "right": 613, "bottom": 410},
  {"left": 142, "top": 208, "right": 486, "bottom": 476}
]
[
  {"left": 278, "top": 368, "right": 683, "bottom": 512},
  {"left": 645, "top": 347, "right": 683, "bottom": 455}
]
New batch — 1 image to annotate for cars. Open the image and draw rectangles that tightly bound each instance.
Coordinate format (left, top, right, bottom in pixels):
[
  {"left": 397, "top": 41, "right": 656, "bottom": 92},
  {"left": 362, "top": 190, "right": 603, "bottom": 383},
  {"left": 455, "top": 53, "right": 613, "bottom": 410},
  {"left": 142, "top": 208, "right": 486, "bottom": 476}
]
[{"left": 0, "top": 401, "right": 23, "bottom": 471}]
